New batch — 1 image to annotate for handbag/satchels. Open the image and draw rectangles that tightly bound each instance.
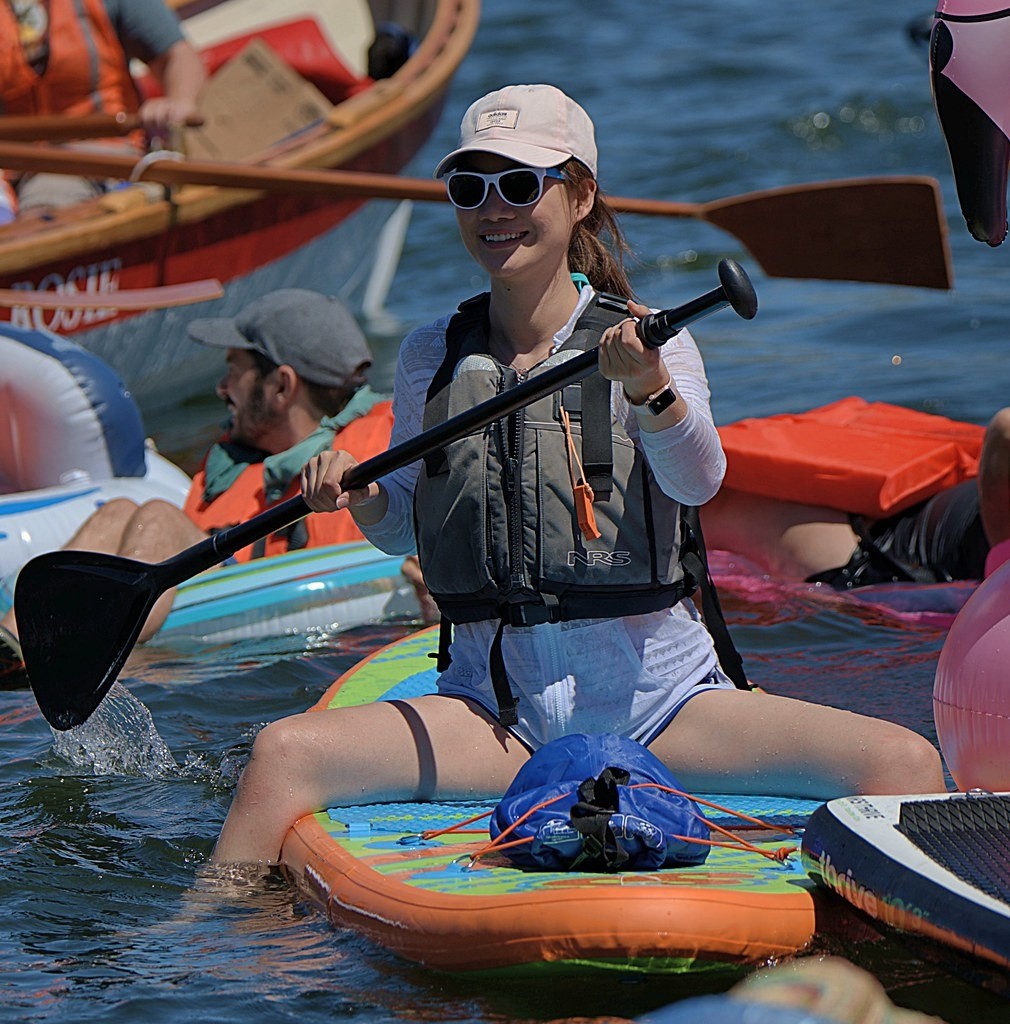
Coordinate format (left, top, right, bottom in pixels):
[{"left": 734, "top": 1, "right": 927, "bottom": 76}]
[{"left": 490, "top": 732, "right": 711, "bottom": 866}]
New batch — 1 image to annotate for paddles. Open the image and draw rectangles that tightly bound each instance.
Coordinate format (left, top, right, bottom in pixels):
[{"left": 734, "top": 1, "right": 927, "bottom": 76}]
[
  {"left": 16, "top": 260, "right": 757, "bottom": 730},
  {"left": 0, "top": 142, "right": 954, "bottom": 297},
  {"left": 0, "top": 114, "right": 208, "bottom": 139}
]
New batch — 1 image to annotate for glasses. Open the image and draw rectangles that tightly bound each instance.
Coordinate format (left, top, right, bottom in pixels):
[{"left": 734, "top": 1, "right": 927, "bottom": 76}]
[{"left": 445, "top": 168, "right": 566, "bottom": 210}]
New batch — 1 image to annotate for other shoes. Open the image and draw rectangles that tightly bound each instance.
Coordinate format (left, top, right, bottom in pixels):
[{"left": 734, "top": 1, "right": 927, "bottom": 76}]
[{"left": 0, "top": 625, "right": 29, "bottom": 696}]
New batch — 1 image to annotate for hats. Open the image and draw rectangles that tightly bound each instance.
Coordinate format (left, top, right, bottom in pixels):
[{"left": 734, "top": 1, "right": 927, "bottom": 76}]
[
  {"left": 188, "top": 288, "right": 371, "bottom": 386},
  {"left": 433, "top": 85, "right": 597, "bottom": 180}
]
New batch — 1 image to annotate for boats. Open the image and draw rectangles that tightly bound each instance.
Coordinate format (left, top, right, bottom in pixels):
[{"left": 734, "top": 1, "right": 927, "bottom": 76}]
[{"left": 0, "top": 1, "right": 484, "bottom": 336}]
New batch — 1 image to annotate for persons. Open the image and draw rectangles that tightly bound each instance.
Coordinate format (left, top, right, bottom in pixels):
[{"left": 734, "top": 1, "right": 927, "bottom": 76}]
[
  {"left": 694, "top": 407, "right": 1010, "bottom": 596},
  {"left": 0, "top": 0, "right": 207, "bottom": 225},
  {"left": 207, "top": 85, "right": 950, "bottom": 862},
  {"left": 0, "top": 289, "right": 397, "bottom": 694}
]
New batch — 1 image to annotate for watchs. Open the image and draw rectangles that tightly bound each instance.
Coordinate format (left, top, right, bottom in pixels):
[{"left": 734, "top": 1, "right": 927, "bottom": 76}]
[{"left": 622, "top": 378, "right": 680, "bottom": 421}]
